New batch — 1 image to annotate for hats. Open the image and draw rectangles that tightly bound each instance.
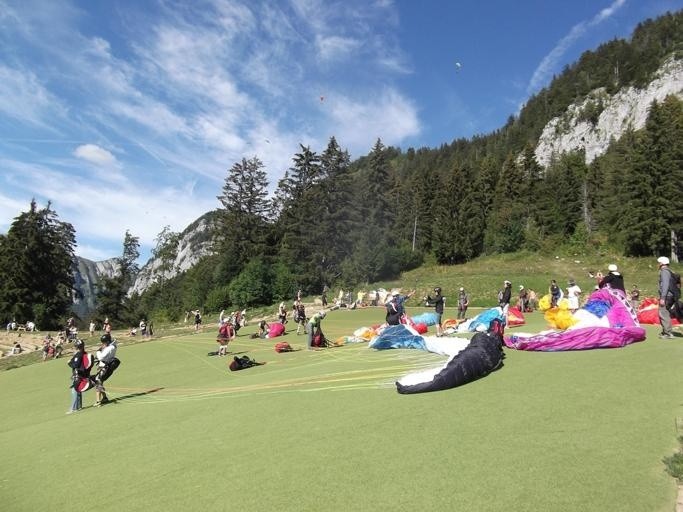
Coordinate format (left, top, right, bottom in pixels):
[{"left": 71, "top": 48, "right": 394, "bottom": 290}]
[
  {"left": 460, "top": 287, "right": 464, "bottom": 292},
  {"left": 567, "top": 279, "right": 576, "bottom": 284},
  {"left": 434, "top": 287, "right": 441, "bottom": 292}
]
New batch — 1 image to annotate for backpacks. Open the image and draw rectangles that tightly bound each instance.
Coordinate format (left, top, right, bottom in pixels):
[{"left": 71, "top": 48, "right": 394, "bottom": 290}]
[
  {"left": 70, "top": 353, "right": 98, "bottom": 392},
  {"left": 659, "top": 269, "right": 681, "bottom": 301}
]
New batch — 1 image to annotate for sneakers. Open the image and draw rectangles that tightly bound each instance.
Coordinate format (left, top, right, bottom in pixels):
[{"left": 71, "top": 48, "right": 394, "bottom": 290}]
[
  {"left": 101, "top": 398, "right": 108, "bottom": 404},
  {"left": 66, "top": 409, "right": 77, "bottom": 414},
  {"left": 93, "top": 401, "right": 101, "bottom": 407},
  {"left": 77, "top": 408, "right": 82, "bottom": 411},
  {"left": 659, "top": 334, "right": 672, "bottom": 339}
]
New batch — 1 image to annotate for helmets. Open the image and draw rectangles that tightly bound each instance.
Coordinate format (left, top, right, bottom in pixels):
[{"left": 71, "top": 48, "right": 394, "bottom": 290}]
[
  {"left": 657, "top": 256, "right": 669, "bottom": 265},
  {"left": 74, "top": 339, "right": 84, "bottom": 347},
  {"left": 392, "top": 291, "right": 400, "bottom": 296},
  {"left": 608, "top": 265, "right": 617, "bottom": 271},
  {"left": 320, "top": 311, "right": 326, "bottom": 319},
  {"left": 101, "top": 335, "right": 111, "bottom": 342}
]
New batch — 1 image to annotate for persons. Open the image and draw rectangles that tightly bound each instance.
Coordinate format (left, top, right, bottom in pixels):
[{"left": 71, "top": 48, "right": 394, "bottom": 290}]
[
  {"left": 88, "top": 318, "right": 97, "bottom": 337},
  {"left": 102, "top": 317, "right": 115, "bottom": 336},
  {"left": 497, "top": 280, "right": 512, "bottom": 329},
  {"left": 385, "top": 311, "right": 401, "bottom": 325},
  {"left": 65, "top": 338, "right": 88, "bottom": 415},
  {"left": 423, "top": 287, "right": 444, "bottom": 338},
  {"left": 306, "top": 310, "right": 326, "bottom": 351},
  {"left": 278, "top": 288, "right": 308, "bottom": 335},
  {"left": 257, "top": 319, "right": 270, "bottom": 337},
  {"left": 93, "top": 333, "right": 117, "bottom": 407},
  {"left": 139, "top": 318, "right": 147, "bottom": 334},
  {"left": 588, "top": 270, "right": 606, "bottom": 288},
  {"left": 528, "top": 287, "right": 537, "bottom": 310},
  {"left": 549, "top": 279, "right": 561, "bottom": 309},
  {"left": 215, "top": 308, "right": 249, "bottom": 356},
  {"left": 6, "top": 314, "right": 79, "bottom": 358},
  {"left": 129, "top": 326, "right": 137, "bottom": 337},
  {"left": 191, "top": 309, "right": 201, "bottom": 331},
  {"left": 146, "top": 320, "right": 155, "bottom": 335},
  {"left": 630, "top": 284, "right": 640, "bottom": 312},
  {"left": 383, "top": 289, "right": 418, "bottom": 314},
  {"left": 566, "top": 279, "right": 582, "bottom": 313},
  {"left": 599, "top": 264, "right": 627, "bottom": 291},
  {"left": 321, "top": 287, "right": 407, "bottom": 309},
  {"left": 654, "top": 256, "right": 674, "bottom": 339},
  {"left": 519, "top": 285, "right": 527, "bottom": 312},
  {"left": 456, "top": 287, "right": 470, "bottom": 319}
]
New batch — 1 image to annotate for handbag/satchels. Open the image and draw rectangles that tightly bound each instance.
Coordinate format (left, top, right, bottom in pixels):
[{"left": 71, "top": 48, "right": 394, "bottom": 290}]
[{"left": 99, "top": 357, "right": 120, "bottom": 382}]
